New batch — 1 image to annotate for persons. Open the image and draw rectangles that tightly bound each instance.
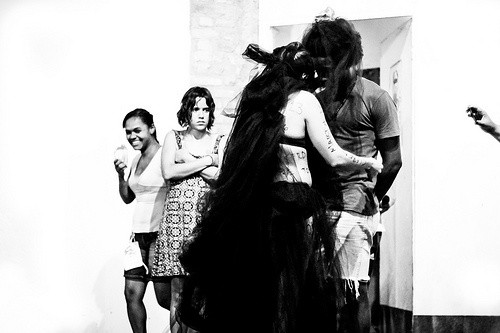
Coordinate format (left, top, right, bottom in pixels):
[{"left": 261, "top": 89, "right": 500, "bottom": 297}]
[
  {"left": 466, "top": 107, "right": 500, "bottom": 149},
  {"left": 163, "top": 87, "right": 229, "bottom": 333},
  {"left": 169, "top": 7, "right": 402, "bottom": 333},
  {"left": 115, "top": 108, "right": 171, "bottom": 333}
]
